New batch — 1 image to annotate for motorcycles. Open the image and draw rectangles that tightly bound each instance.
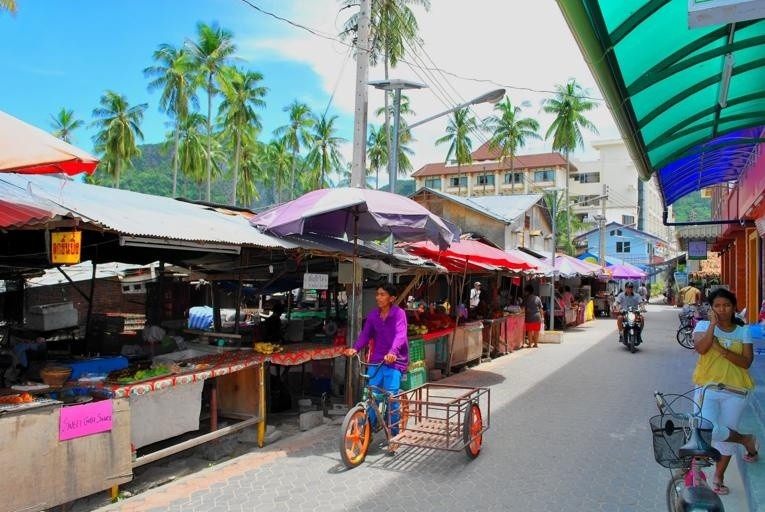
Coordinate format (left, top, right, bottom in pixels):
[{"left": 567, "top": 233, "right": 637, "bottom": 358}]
[{"left": 612, "top": 306, "right": 647, "bottom": 352}]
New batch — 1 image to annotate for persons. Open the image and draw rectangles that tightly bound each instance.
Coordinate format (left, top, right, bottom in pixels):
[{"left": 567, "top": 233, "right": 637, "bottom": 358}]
[
  {"left": 520, "top": 284, "right": 545, "bottom": 348},
  {"left": 637, "top": 282, "right": 648, "bottom": 313},
  {"left": 611, "top": 281, "right": 647, "bottom": 344},
  {"left": 403, "top": 280, "right": 524, "bottom": 325},
  {"left": 678, "top": 281, "right": 702, "bottom": 327},
  {"left": 554, "top": 284, "right": 588, "bottom": 329},
  {"left": 340, "top": 282, "right": 408, "bottom": 438},
  {"left": 687, "top": 287, "right": 762, "bottom": 495}
]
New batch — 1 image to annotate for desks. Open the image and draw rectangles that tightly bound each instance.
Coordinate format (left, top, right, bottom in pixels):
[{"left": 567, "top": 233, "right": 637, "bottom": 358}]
[
  {"left": 365, "top": 299, "right": 527, "bottom": 376},
  {"left": 62, "top": 342, "right": 346, "bottom": 469},
  {"left": 0, "top": 398, "right": 133, "bottom": 512}
]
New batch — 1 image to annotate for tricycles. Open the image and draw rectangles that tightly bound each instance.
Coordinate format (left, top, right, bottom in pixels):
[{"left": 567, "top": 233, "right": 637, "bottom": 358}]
[{"left": 339, "top": 349, "right": 490, "bottom": 468}]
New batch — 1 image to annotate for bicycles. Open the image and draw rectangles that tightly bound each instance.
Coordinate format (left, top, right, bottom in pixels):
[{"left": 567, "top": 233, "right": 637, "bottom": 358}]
[
  {"left": 650, "top": 383, "right": 748, "bottom": 512},
  {"left": 676, "top": 302, "right": 711, "bottom": 349},
  {"left": 663, "top": 292, "right": 671, "bottom": 305}
]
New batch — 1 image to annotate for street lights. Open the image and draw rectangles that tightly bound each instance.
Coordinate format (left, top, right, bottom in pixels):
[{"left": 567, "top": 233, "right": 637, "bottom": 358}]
[
  {"left": 368, "top": 79, "right": 506, "bottom": 193},
  {"left": 543, "top": 186, "right": 656, "bottom": 331}
]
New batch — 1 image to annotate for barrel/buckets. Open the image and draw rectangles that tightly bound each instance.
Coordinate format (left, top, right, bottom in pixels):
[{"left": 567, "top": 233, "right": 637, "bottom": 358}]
[{"left": 287, "top": 320, "right": 305, "bottom": 342}]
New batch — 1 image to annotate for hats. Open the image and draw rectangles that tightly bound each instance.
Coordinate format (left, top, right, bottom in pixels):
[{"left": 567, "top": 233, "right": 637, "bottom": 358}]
[
  {"left": 625, "top": 281, "right": 634, "bottom": 287},
  {"left": 473, "top": 281, "right": 482, "bottom": 286}
]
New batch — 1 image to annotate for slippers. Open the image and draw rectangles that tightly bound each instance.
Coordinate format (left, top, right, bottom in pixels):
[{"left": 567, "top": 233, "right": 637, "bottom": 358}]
[
  {"left": 740, "top": 439, "right": 761, "bottom": 463},
  {"left": 711, "top": 480, "right": 728, "bottom": 494}
]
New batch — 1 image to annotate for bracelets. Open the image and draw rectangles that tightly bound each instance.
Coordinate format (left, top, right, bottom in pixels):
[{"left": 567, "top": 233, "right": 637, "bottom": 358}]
[{"left": 723, "top": 350, "right": 730, "bottom": 358}]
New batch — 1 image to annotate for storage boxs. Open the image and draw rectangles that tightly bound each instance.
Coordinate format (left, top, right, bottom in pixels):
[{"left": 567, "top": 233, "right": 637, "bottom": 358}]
[
  {"left": 539, "top": 331, "right": 563, "bottom": 343},
  {"left": 27, "top": 303, "right": 78, "bottom": 331}
]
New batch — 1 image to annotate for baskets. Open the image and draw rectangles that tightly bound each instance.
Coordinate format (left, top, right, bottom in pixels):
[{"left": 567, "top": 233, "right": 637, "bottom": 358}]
[
  {"left": 407, "top": 338, "right": 427, "bottom": 363},
  {"left": 398, "top": 367, "right": 427, "bottom": 392}
]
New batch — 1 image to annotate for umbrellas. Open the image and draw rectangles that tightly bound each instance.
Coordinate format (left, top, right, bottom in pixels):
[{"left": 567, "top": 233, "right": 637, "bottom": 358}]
[
  {"left": 404, "top": 235, "right": 536, "bottom": 373},
  {"left": 0, "top": 109, "right": 96, "bottom": 179},
  {"left": 249, "top": 184, "right": 457, "bottom": 409},
  {"left": 502, "top": 246, "right": 649, "bottom": 305}
]
